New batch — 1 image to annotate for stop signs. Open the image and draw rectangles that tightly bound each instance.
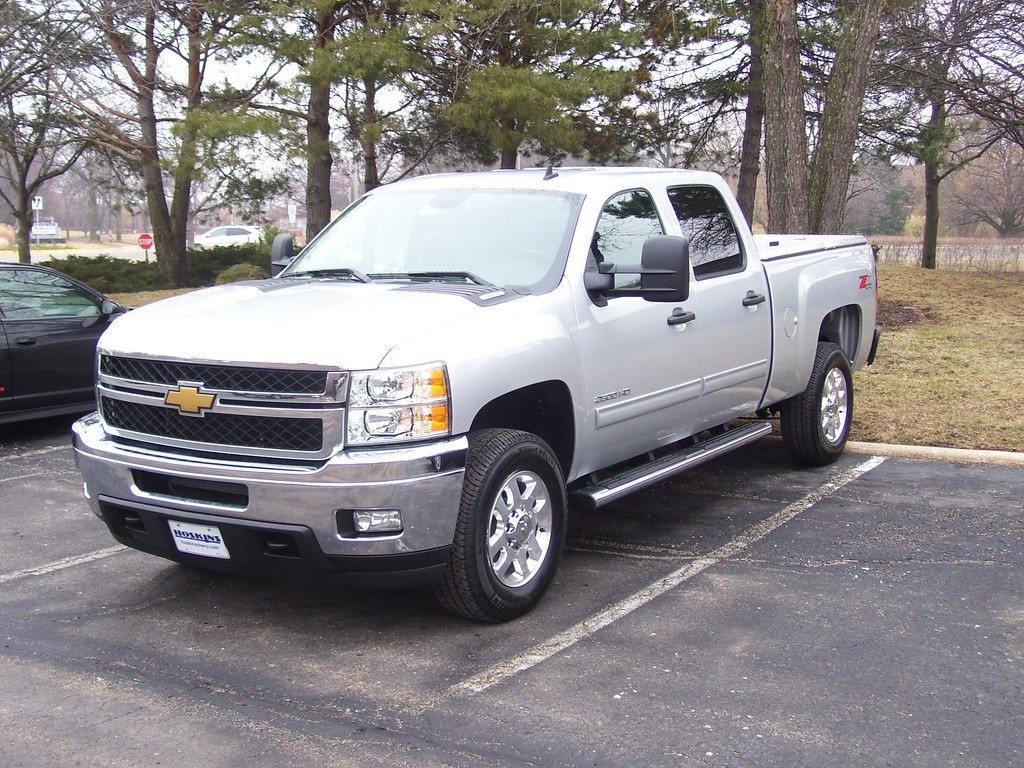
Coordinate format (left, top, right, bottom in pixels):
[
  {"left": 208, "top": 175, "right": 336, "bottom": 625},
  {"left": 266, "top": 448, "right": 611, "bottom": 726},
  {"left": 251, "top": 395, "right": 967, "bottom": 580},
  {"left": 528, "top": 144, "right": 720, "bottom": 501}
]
[{"left": 138, "top": 234, "right": 153, "bottom": 249}]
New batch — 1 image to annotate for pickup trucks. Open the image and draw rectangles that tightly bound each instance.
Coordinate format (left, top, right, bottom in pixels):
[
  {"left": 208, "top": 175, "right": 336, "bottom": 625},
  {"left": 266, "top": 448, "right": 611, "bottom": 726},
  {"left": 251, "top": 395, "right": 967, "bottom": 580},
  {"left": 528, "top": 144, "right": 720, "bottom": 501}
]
[{"left": 69, "top": 165, "right": 885, "bottom": 627}]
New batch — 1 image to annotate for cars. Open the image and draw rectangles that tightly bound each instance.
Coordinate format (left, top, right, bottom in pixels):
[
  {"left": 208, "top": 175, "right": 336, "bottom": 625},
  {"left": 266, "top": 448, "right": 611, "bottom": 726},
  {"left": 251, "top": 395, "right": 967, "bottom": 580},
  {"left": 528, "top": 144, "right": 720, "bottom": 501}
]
[
  {"left": 0, "top": 260, "right": 140, "bottom": 427},
  {"left": 185, "top": 225, "right": 264, "bottom": 250}
]
